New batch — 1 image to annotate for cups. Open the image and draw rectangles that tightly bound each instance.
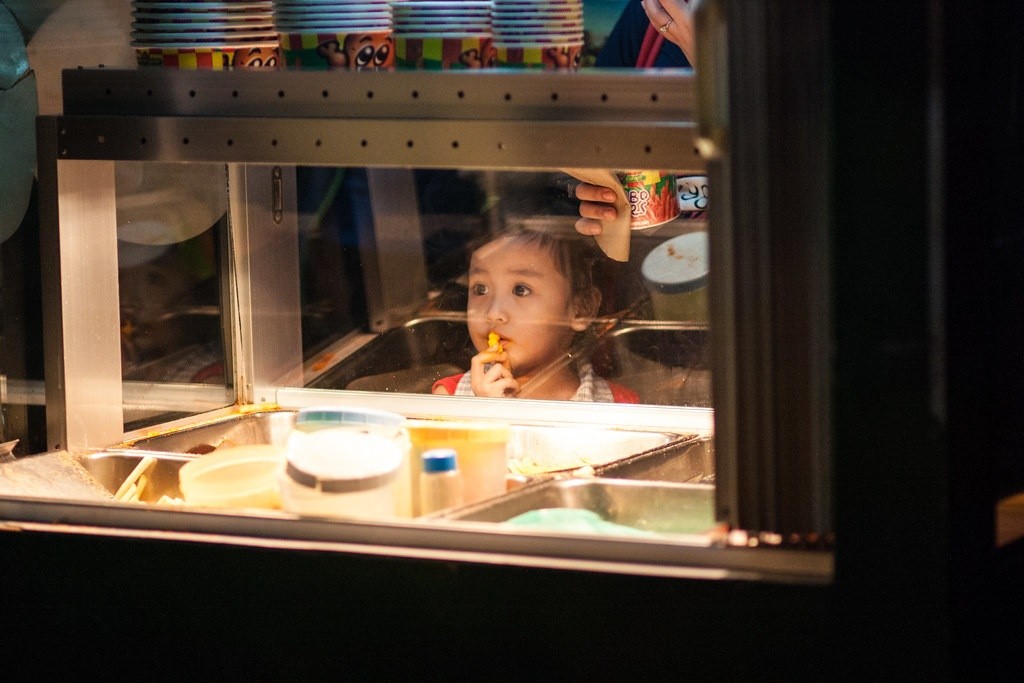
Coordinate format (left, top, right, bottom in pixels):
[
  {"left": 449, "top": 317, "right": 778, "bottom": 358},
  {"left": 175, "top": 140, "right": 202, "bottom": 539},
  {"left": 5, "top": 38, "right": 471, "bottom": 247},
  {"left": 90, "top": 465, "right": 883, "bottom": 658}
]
[
  {"left": 127, "top": 0, "right": 585, "bottom": 72},
  {"left": 180, "top": 444, "right": 286, "bottom": 512}
]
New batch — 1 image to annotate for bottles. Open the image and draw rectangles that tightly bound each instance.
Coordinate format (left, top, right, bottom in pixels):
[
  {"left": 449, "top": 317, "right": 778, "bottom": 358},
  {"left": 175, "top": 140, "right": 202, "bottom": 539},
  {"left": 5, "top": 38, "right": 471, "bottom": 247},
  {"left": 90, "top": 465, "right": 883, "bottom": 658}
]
[
  {"left": 420, "top": 451, "right": 462, "bottom": 516},
  {"left": 408, "top": 418, "right": 512, "bottom": 517},
  {"left": 640, "top": 230, "right": 709, "bottom": 324},
  {"left": 283, "top": 424, "right": 405, "bottom": 523}
]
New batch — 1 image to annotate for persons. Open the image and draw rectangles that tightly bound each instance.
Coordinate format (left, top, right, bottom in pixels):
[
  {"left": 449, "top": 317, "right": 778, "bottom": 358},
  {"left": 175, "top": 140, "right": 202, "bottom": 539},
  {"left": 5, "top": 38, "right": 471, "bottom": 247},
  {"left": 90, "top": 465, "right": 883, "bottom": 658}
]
[{"left": 431, "top": 0, "right": 711, "bottom": 405}]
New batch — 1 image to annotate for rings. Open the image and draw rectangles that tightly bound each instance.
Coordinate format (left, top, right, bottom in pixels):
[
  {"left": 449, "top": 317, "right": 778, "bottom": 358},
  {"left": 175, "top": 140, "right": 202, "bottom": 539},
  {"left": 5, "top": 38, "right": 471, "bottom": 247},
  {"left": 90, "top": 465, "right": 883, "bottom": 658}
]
[{"left": 659, "top": 19, "right": 673, "bottom": 32}]
